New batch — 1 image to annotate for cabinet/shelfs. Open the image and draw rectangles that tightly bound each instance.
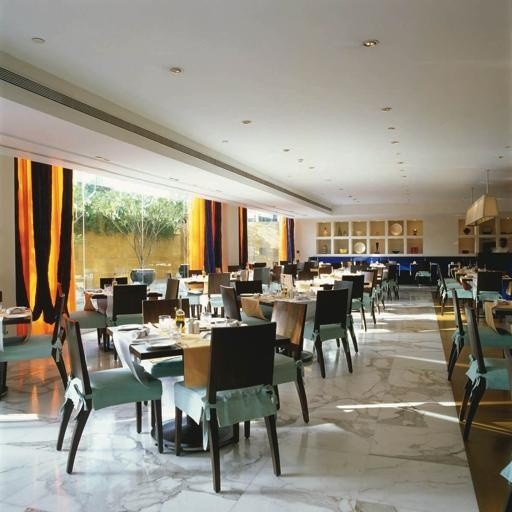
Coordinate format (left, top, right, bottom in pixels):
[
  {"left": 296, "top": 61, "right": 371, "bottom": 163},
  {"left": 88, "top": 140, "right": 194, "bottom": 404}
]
[
  {"left": 458, "top": 216, "right": 512, "bottom": 256},
  {"left": 316, "top": 219, "right": 425, "bottom": 256}
]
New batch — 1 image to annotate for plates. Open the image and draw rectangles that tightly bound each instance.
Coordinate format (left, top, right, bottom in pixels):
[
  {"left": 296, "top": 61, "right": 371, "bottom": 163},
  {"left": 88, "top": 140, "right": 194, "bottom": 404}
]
[
  {"left": 353, "top": 242, "right": 365, "bottom": 254},
  {"left": 389, "top": 223, "right": 402, "bottom": 235},
  {"left": 116, "top": 304, "right": 248, "bottom": 349}
]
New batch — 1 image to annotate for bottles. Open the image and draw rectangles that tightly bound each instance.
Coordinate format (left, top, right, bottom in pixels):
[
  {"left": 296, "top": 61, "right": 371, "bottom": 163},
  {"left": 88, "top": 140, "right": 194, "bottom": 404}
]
[
  {"left": 201, "top": 265, "right": 206, "bottom": 279},
  {"left": 112, "top": 272, "right": 118, "bottom": 288}
]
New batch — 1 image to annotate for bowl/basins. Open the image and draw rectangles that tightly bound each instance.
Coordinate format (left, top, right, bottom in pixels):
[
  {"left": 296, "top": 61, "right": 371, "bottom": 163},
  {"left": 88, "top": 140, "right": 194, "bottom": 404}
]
[
  {"left": 392, "top": 250, "right": 399, "bottom": 253},
  {"left": 251, "top": 277, "right": 317, "bottom": 300},
  {"left": 339, "top": 248, "right": 347, "bottom": 254}
]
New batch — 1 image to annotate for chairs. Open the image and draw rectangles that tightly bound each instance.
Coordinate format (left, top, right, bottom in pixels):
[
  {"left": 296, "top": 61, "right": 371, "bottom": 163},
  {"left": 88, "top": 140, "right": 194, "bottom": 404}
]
[
  {"left": 113, "top": 284, "right": 148, "bottom": 362},
  {"left": 57, "top": 284, "right": 108, "bottom": 354},
  {"left": 431, "top": 257, "right": 512, "bottom": 512},
  {"left": 242, "top": 300, "right": 308, "bottom": 437},
  {"left": 302, "top": 290, "right": 353, "bottom": 378},
  {"left": 174, "top": 320, "right": 279, "bottom": 499},
  {"left": 58, "top": 314, "right": 164, "bottom": 476},
  {"left": 85, "top": 255, "right": 402, "bottom": 353},
  {"left": 140, "top": 299, "right": 182, "bottom": 433},
  {"left": 2, "top": 295, "right": 64, "bottom": 397}
]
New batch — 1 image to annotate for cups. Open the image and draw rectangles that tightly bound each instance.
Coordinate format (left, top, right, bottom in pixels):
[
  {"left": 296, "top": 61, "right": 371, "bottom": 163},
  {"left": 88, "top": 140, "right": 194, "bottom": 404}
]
[{"left": 497, "top": 299, "right": 510, "bottom": 305}]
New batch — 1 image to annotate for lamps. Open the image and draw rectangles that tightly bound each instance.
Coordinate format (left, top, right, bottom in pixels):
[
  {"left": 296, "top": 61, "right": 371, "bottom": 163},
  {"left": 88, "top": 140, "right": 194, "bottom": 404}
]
[{"left": 465, "top": 169, "right": 498, "bottom": 230}]
